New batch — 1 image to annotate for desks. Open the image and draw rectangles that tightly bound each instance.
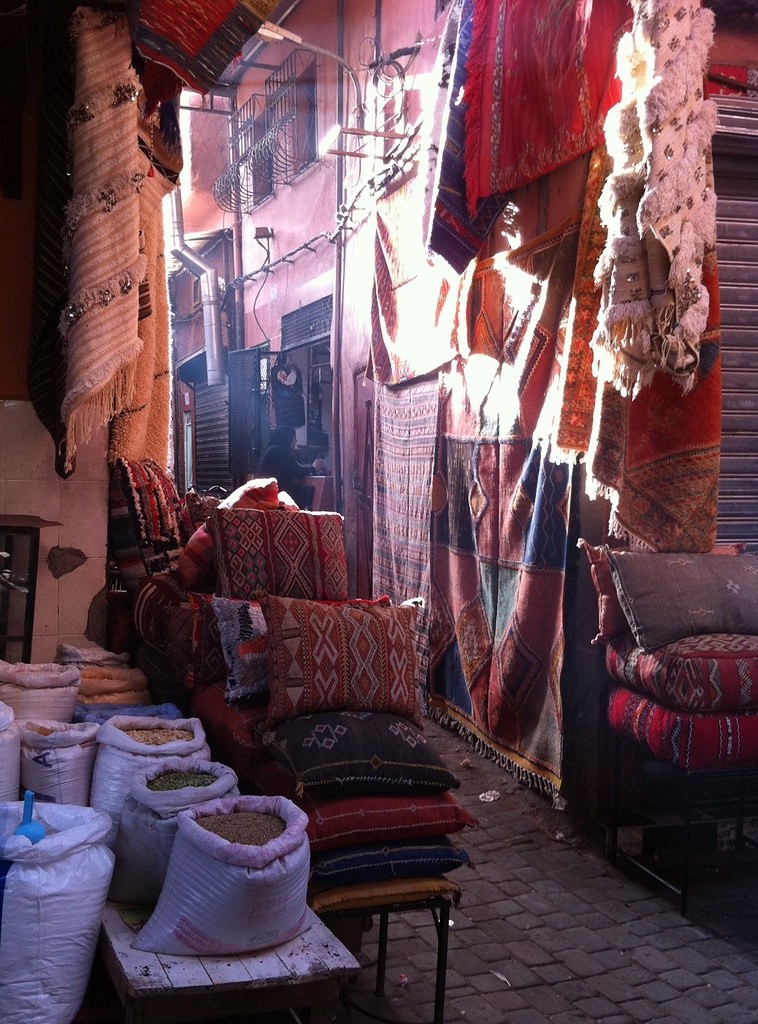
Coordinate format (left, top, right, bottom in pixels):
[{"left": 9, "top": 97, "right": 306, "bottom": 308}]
[{"left": 95, "top": 900, "right": 361, "bottom": 1024}]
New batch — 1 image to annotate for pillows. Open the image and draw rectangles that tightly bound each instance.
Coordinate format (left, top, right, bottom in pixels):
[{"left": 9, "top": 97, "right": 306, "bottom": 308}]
[
  {"left": 206, "top": 506, "right": 349, "bottom": 601},
  {"left": 603, "top": 544, "right": 758, "bottom": 655},
  {"left": 261, "top": 713, "right": 478, "bottom": 879},
  {"left": 177, "top": 477, "right": 280, "bottom": 584},
  {"left": 187, "top": 590, "right": 392, "bottom": 695},
  {"left": 211, "top": 592, "right": 270, "bottom": 710},
  {"left": 253, "top": 589, "right": 426, "bottom": 733},
  {"left": 576, "top": 537, "right": 747, "bottom": 618}
]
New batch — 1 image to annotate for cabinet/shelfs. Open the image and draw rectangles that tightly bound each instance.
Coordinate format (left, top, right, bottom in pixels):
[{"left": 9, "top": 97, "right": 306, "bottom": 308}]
[
  {"left": 606, "top": 734, "right": 757, "bottom": 917},
  {"left": 315, "top": 894, "right": 452, "bottom": 1024}
]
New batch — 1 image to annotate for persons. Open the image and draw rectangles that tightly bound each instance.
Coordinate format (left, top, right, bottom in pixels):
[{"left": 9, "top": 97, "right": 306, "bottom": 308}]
[{"left": 256, "top": 424, "right": 323, "bottom": 512}]
